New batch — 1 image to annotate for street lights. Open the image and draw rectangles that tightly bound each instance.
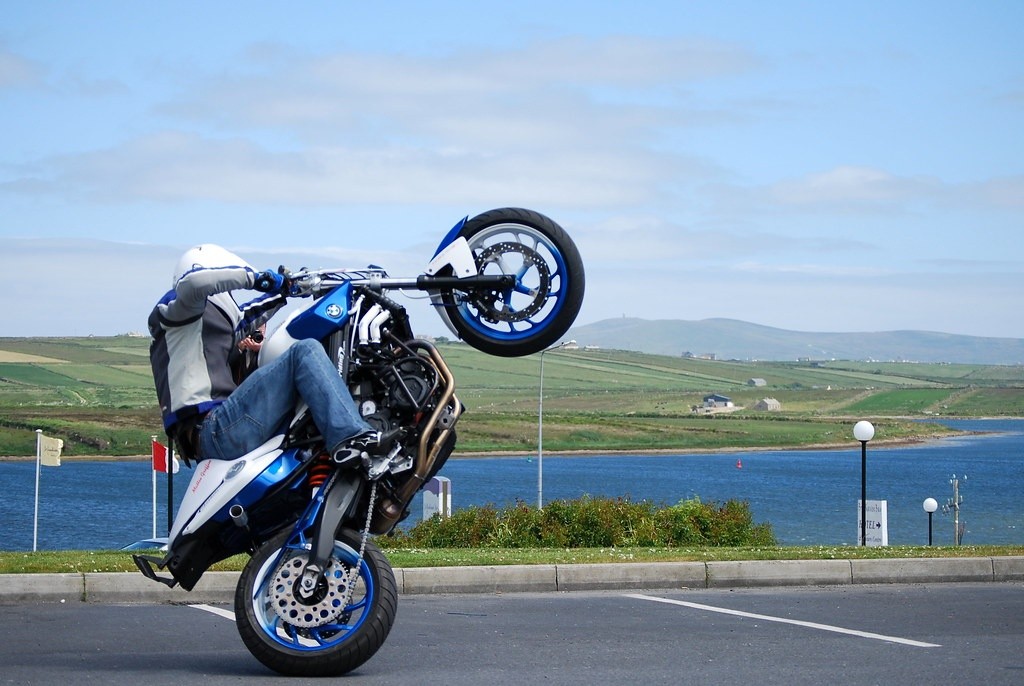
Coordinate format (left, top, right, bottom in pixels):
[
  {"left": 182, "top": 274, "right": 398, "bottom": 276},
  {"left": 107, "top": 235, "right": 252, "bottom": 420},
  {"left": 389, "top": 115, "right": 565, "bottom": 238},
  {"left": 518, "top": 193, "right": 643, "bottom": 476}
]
[
  {"left": 853, "top": 421, "right": 876, "bottom": 548},
  {"left": 537, "top": 340, "right": 577, "bottom": 512},
  {"left": 923, "top": 499, "right": 938, "bottom": 546}
]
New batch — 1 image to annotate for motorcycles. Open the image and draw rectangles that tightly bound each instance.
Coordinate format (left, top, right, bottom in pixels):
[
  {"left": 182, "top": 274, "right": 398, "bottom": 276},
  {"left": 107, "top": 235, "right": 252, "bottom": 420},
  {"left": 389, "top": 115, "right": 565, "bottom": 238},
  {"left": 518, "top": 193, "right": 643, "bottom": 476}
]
[{"left": 116, "top": 208, "right": 585, "bottom": 677}]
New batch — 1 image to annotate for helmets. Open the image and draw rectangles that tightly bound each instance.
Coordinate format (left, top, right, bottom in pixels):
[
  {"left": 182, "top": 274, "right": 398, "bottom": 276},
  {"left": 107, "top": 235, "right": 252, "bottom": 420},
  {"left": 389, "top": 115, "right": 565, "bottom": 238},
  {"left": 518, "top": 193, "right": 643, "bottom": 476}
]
[{"left": 172, "top": 243, "right": 256, "bottom": 335}]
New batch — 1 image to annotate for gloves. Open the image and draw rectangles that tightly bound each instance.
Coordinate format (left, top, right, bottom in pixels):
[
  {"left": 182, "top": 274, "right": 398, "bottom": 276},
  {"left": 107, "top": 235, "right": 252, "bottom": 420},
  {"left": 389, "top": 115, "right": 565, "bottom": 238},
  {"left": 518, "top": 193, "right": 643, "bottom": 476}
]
[{"left": 254, "top": 269, "right": 287, "bottom": 292}]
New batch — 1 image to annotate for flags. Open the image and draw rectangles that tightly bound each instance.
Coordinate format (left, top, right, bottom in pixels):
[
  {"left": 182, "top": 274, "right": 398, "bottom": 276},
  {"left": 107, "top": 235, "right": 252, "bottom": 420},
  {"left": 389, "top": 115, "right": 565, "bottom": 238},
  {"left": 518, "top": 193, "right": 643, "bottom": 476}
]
[
  {"left": 41, "top": 434, "right": 63, "bottom": 467},
  {"left": 153, "top": 444, "right": 180, "bottom": 474}
]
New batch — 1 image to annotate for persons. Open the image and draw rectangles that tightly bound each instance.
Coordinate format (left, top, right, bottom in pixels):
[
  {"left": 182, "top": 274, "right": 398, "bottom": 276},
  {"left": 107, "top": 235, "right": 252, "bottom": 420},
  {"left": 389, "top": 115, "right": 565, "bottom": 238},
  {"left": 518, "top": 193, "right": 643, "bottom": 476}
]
[{"left": 147, "top": 244, "right": 403, "bottom": 470}]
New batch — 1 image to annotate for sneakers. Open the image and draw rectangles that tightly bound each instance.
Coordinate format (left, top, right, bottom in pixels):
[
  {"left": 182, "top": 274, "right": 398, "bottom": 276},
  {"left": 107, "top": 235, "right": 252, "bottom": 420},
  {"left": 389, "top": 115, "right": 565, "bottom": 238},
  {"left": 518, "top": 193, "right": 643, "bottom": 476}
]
[{"left": 330, "top": 427, "right": 402, "bottom": 466}]
